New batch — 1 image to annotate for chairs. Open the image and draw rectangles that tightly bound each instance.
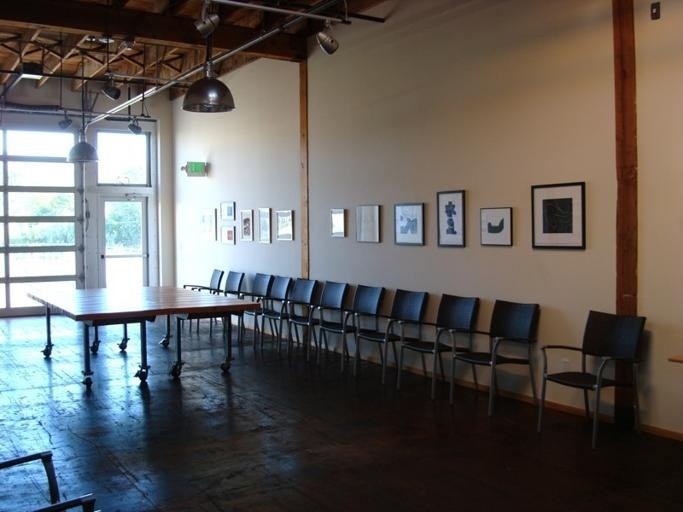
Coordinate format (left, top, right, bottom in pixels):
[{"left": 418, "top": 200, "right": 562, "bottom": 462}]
[
  {"left": 189, "top": 270, "right": 349, "bottom": 361},
  {"left": 316, "top": 284, "right": 385, "bottom": 374},
  {"left": 354, "top": 288, "right": 429, "bottom": 387},
  {"left": 397, "top": 292, "right": 480, "bottom": 405},
  {"left": 543, "top": 310, "right": 646, "bottom": 444},
  {"left": 0, "top": 451, "right": 95, "bottom": 509},
  {"left": 448, "top": 299, "right": 539, "bottom": 415}
]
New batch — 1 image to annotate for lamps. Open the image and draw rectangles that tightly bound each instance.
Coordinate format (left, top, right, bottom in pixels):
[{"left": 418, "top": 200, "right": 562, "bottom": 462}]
[
  {"left": 13, "top": 37, "right": 44, "bottom": 79},
  {"left": 190, "top": 1, "right": 348, "bottom": 55},
  {"left": 181, "top": 29, "right": 236, "bottom": 115},
  {"left": 55, "top": 109, "right": 151, "bottom": 134},
  {"left": 67, "top": 54, "right": 98, "bottom": 166},
  {"left": 98, "top": 36, "right": 122, "bottom": 99}
]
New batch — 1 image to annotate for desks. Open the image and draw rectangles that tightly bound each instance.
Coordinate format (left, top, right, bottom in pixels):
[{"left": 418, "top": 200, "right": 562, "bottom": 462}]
[{"left": 28, "top": 286, "right": 262, "bottom": 391}]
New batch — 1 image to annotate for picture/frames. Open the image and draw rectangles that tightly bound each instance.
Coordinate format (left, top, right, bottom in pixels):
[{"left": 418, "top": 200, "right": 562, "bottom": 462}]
[
  {"left": 354, "top": 205, "right": 380, "bottom": 242},
  {"left": 478, "top": 207, "right": 514, "bottom": 248},
  {"left": 435, "top": 189, "right": 466, "bottom": 249},
  {"left": 390, "top": 201, "right": 426, "bottom": 247},
  {"left": 327, "top": 206, "right": 345, "bottom": 239},
  {"left": 212, "top": 190, "right": 295, "bottom": 246},
  {"left": 528, "top": 182, "right": 586, "bottom": 253}
]
